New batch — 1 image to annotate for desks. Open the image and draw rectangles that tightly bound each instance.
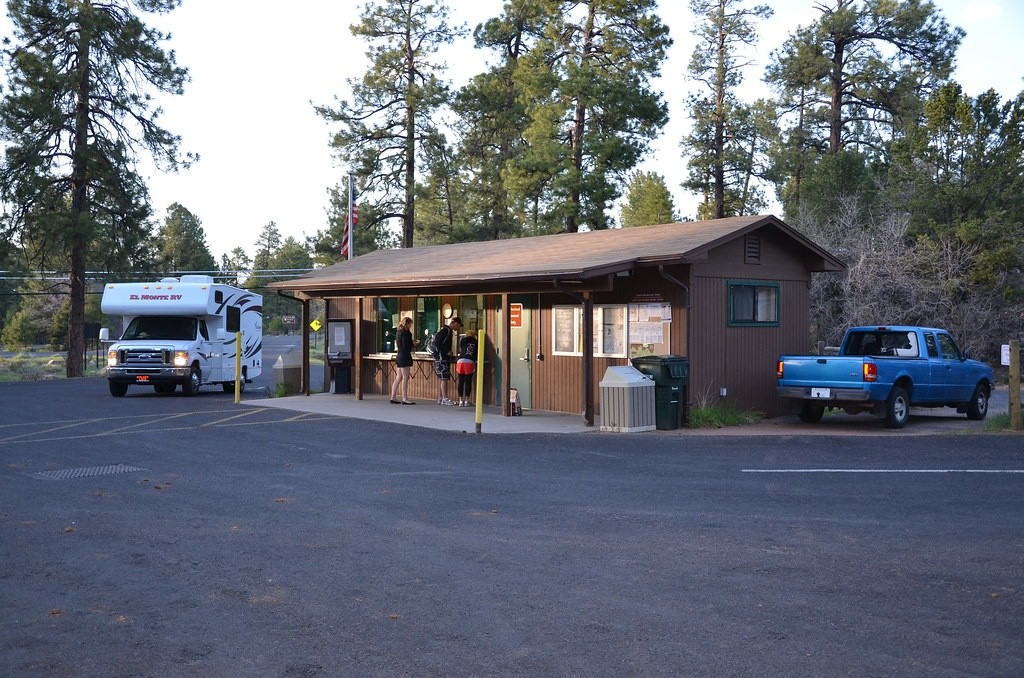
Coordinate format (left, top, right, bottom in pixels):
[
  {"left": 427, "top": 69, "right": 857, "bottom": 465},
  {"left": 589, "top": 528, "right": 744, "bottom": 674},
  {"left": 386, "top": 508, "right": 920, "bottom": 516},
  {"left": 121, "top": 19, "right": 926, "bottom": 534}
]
[{"left": 363, "top": 353, "right": 489, "bottom": 382}]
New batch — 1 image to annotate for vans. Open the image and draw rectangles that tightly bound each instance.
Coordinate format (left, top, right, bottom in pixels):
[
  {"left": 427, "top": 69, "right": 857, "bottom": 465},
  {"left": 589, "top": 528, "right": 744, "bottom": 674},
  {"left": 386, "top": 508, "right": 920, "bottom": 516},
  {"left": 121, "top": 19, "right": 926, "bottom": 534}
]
[{"left": 101, "top": 275, "right": 263, "bottom": 397}]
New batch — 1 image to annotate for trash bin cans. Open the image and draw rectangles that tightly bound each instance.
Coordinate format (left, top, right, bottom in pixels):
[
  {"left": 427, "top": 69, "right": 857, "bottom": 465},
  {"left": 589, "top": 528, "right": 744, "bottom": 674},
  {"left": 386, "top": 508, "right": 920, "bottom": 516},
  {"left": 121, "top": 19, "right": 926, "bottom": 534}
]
[
  {"left": 631, "top": 354, "right": 695, "bottom": 432},
  {"left": 597, "top": 366, "right": 656, "bottom": 434},
  {"left": 272, "top": 354, "right": 303, "bottom": 395}
]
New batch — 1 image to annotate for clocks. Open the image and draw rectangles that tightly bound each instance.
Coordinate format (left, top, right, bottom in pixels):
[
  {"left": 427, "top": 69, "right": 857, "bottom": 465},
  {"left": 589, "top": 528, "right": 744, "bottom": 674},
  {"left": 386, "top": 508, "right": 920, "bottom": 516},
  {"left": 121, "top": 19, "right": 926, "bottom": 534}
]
[{"left": 442, "top": 303, "right": 453, "bottom": 319}]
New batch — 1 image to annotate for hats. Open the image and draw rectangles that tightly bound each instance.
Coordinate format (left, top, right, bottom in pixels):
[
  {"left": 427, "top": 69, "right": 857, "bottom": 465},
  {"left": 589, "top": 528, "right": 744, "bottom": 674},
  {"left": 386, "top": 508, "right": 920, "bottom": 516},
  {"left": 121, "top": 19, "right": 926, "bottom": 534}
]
[{"left": 452, "top": 317, "right": 464, "bottom": 328}]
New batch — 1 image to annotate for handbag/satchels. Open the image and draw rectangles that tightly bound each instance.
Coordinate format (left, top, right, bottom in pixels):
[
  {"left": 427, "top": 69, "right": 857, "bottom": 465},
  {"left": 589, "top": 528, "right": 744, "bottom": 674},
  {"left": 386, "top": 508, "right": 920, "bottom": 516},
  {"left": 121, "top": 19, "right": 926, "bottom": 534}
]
[{"left": 426, "top": 341, "right": 438, "bottom": 356}]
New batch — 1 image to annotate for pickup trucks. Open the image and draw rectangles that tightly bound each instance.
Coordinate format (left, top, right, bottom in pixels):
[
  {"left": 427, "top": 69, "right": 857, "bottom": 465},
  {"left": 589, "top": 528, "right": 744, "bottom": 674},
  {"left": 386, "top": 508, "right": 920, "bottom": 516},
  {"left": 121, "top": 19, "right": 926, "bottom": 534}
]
[{"left": 774, "top": 326, "right": 993, "bottom": 429}]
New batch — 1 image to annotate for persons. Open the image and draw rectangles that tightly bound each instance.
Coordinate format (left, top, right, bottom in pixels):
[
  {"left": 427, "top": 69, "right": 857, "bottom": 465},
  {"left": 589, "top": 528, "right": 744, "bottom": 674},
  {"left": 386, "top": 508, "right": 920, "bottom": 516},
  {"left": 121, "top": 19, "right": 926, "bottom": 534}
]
[
  {"left": 456, "top": 330, "right": 478, "bottom": 407},
  {"left": 390, "top": 317, "right": 418, "bottom": 405},
  {"left": 431, "top": 317, "right": 464, "bottom": 406},
  {"left": 284, "top": 325, "right": 290, "bottom": 336}
]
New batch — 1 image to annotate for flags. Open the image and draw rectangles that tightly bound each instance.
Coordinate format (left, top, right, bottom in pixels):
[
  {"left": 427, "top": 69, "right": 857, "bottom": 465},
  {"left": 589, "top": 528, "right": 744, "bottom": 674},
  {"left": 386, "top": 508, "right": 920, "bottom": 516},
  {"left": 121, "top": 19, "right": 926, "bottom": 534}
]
[{"left": 341, "top": 183, "right": 358, "bottom": 257}]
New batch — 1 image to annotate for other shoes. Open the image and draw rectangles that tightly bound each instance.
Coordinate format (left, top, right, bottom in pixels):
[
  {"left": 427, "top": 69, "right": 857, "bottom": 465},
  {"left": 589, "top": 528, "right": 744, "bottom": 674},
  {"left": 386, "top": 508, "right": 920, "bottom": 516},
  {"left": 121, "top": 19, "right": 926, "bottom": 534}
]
[
  {"left": 437, "top": 398, "right": 443, "bottom": 404},
  {"left": 458, "top": 401, "right": 463, "bottom": 407},
  {"left": 390, "top": 400, "right": 401, "bottom": 404},
  {"left": 402, "top": 401, "right": 416, "bottom": 405},
  {"left": 441, "top": 398, "right": 455, "bottom": 406},
  {"left": 464, "top": 400, "right": 472, "bottom": 406}
]
